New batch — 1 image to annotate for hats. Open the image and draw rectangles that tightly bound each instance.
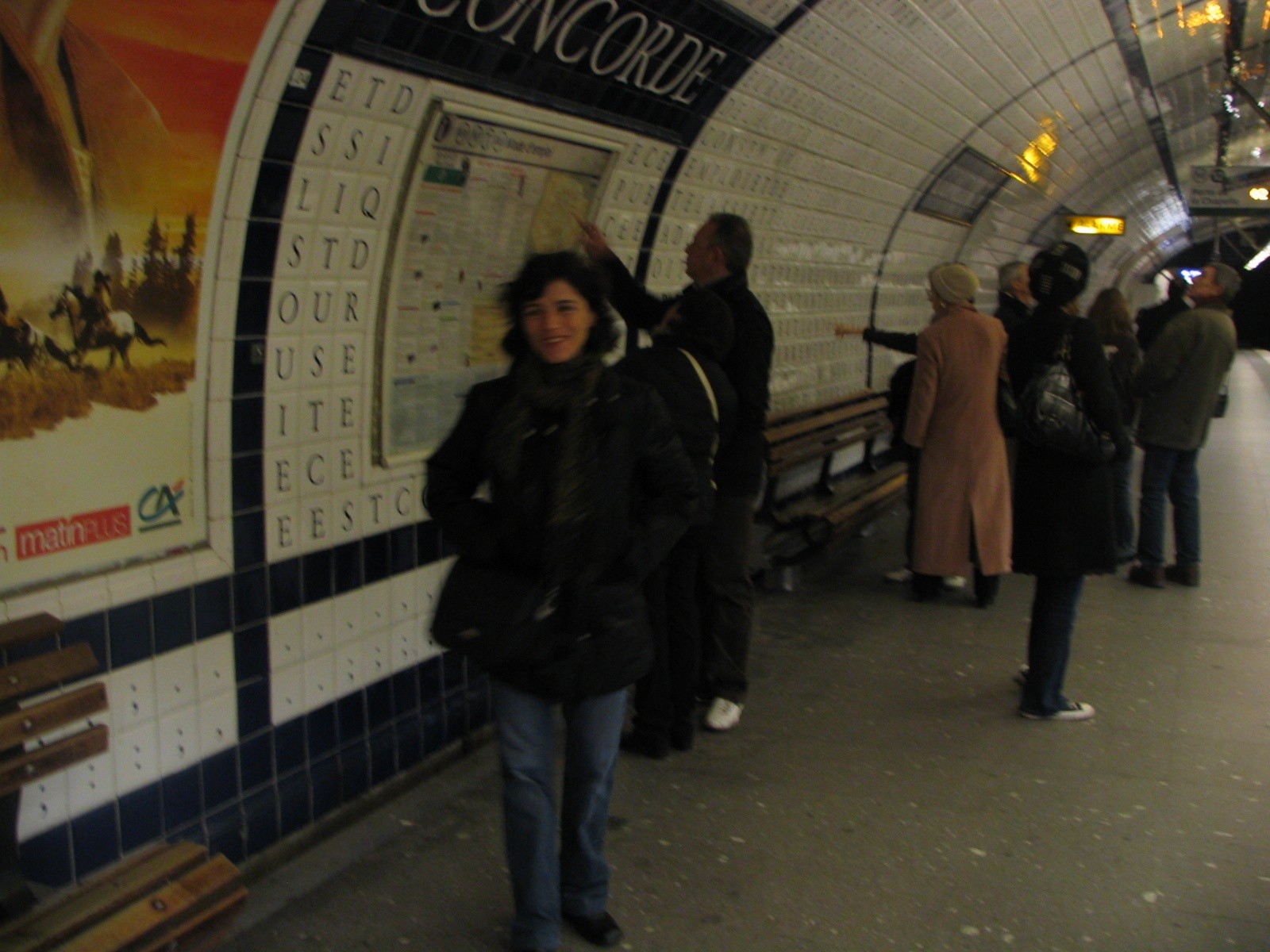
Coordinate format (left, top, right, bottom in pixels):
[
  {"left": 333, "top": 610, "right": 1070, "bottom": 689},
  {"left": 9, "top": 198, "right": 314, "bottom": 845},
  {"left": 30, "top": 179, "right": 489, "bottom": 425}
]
[
  {"left": 1029, "top": 241, "right": 1087, "bottom": 301},
  {"left": 929, "top": 260, "right": 980, "bottom": 304}
]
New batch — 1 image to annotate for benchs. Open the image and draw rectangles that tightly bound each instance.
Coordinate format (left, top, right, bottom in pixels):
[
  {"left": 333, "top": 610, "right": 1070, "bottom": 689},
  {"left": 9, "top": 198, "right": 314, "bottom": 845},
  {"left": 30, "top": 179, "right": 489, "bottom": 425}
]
[
  {"left": 0, "top": 611, "right": 249, "bottom": 952},
  {"left": 755, "top": 387, "right": 909, "bottom": 597}
]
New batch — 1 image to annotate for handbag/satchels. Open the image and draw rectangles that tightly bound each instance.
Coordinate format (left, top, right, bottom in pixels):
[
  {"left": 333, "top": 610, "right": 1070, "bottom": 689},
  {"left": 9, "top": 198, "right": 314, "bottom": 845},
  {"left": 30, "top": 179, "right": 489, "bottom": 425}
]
[{"left": 432, "top": 507, "right": 530, "bottom": 649}]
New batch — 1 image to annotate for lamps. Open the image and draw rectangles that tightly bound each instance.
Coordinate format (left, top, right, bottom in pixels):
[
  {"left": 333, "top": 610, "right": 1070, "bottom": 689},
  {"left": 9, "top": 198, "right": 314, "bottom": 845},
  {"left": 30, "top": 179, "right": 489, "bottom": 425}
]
[{"left": 1065, "top": 212, "right": 1127, "bottom": 237}]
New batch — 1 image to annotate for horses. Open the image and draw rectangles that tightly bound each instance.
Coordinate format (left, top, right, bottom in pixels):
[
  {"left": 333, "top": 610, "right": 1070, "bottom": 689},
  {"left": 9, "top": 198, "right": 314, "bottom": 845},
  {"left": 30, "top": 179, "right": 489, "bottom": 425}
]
[
  {"left": 50, "top": 285, "right": 166, "bottom": 369},
  {"left": 0, "top": 291, "right": 76, "bottom": 380}
]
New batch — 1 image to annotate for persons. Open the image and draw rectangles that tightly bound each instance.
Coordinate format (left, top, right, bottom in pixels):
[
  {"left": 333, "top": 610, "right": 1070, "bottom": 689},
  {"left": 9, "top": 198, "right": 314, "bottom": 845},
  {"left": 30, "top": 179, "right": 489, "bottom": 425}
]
[
  {"left": 420, "top": 251, "right": 699, "bottom": 952},
  {"left": 834, "top": 262, "right": 1242, "bottom": 609},
  {"left": 83, "top": 271, "right": 113, "bottom": 345},
  {"left": 995, "top": 241, "right": 1134, "bottom": 720},
  {"left": 566, "top": 211, "right": 773, "bottom": 760}
]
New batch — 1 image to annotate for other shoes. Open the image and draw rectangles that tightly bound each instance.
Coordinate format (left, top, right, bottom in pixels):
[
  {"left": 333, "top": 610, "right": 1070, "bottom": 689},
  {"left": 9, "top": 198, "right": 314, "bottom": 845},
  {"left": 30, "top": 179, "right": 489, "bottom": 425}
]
[
  {"left": 938, "top": 572, "right": 966, "bottom": 589},
  {"left": 617, "top": 730, "right": 666, "bottom": 758},
  {"left": 888, "top": 567, "right": 913, "bottom": 582},
  {"left": 562, "top": 904, "right": 621, "bottom": 945},
  {"left": 1130, "top": 565, "right": 1166, "bottom": 590},
  {"left": 1164, "top": 563, "right": 1201, "bottom": 589},
  {"left": 632, "top": 715, "right": 691, "bottom": 750},
  {"left": 707, "top": 697, "right": 743, "bottom": 730}
]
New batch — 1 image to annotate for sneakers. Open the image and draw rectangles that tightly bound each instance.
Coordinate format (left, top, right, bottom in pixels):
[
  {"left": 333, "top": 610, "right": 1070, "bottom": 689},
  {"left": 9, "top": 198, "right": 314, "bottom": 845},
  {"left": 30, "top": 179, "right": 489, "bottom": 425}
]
[{"left": 1020, "top": 697, "right": 1094, "bottom": 721}]
